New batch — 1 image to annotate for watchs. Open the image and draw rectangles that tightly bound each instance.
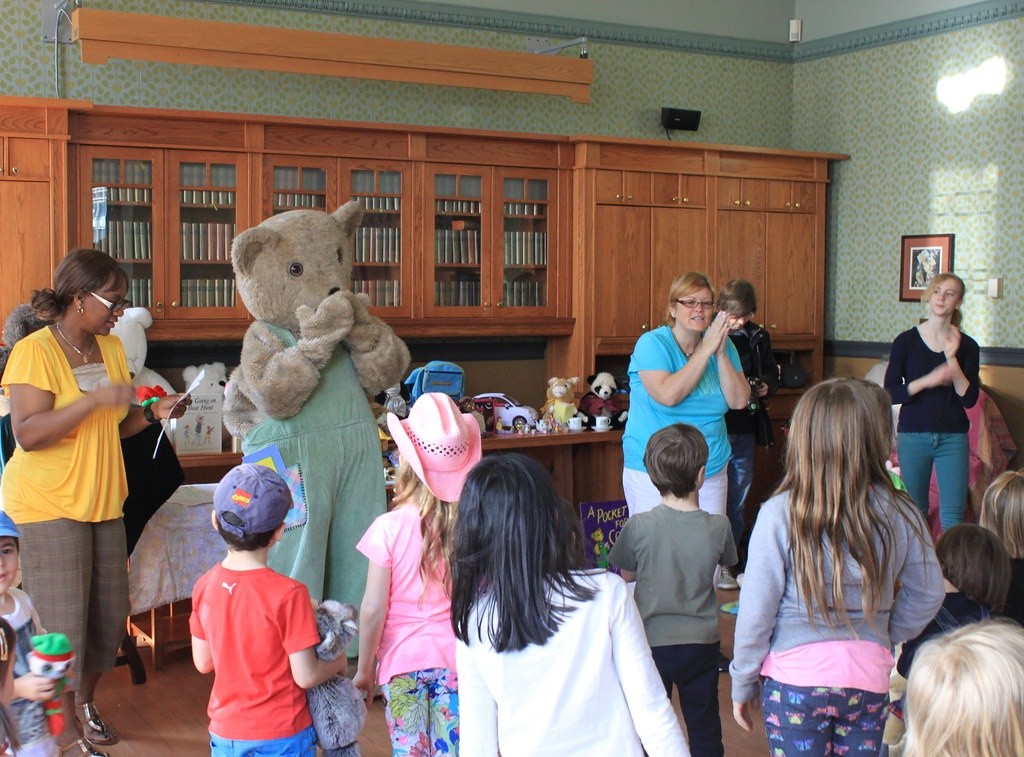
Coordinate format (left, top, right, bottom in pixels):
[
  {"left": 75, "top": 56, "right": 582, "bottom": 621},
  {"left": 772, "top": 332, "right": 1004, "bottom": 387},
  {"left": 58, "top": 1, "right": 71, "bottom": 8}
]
[{"left": 144, "top": 404, "right": 161, "bottom": 423}]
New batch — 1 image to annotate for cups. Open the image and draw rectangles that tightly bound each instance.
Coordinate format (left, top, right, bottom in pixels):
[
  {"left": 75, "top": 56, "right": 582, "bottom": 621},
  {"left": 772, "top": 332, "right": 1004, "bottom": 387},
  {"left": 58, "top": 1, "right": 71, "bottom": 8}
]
[
  {"left": 596, "top": 416, "right": 611, "bottom": 427},
  {"left": 567, "top": 417, "right": 582, "bottom": 428}
]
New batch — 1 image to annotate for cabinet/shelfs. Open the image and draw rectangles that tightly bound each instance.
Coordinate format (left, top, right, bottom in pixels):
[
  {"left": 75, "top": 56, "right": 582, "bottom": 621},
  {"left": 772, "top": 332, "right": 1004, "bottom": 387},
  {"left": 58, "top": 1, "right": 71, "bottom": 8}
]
[{"left": 0, "top": 91, "right": 853, "bottom": 502}]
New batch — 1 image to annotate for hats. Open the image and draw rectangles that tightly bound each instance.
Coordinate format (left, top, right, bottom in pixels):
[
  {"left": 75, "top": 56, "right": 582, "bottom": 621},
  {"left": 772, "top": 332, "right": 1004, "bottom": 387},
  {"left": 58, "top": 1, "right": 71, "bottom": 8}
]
[
  {"left": 212, "top": 464, "right": 293, "bottom": 538},
  {"left": 386, "top": 393, "right": 482, "bottom": 502},
  {"left": 0, "top": 512, "right": 20, "bottom": 538}
]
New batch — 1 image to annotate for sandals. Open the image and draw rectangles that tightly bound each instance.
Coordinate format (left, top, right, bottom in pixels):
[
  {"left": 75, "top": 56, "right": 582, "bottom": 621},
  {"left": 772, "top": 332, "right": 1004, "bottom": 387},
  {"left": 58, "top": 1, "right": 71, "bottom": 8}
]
[
  {"left": 75, "top": 701, "right": 119, "bottom": 745},
  {"left": 61, "top": 738, "right": 111, "bottom": 757}
]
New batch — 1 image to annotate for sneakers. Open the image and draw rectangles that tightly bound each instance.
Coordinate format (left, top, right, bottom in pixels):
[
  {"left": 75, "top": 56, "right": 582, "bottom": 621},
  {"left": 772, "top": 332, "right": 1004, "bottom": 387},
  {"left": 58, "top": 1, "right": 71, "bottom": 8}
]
[{"left": 716, "top": 565, "right": 738, "bottom": 590}]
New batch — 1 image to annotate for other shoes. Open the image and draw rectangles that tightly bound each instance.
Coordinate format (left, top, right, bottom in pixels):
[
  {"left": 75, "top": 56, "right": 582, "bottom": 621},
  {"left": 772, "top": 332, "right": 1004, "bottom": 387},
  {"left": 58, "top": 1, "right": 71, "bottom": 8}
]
[{"left": 717, "top": 654, "right": 731, "bottom": 673}]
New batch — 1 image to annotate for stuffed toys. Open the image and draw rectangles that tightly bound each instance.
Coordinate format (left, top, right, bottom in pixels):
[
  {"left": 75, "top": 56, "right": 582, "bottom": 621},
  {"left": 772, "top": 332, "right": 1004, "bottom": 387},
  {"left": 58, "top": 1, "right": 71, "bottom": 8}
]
[
  {"left": 222, "top": 201, "right": 411, "bottom": 657},
  {"left": 306, "top": 598, "right": 368, "bottom": 757},
  {"left": 110, "top": 307, "right": 177, "bottom": 442},
  {"left": 26, "top": 633, "right": 76, "bottom": 737},
  {"left": 539, "top": 372, "right": 629, "bottom": 430},
  {"left": 182, "top": 362, "right": 227, "bottom": 395},
  {"left": 0, "top": 303, "right": 54, "bottom": 376},
  {"left": 881, "top": 674, "right": 907, "bottom": 745}
]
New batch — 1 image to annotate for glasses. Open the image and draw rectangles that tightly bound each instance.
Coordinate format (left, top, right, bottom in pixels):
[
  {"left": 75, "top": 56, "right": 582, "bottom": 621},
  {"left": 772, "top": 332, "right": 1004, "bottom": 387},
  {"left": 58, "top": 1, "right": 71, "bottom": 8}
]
[
  {"left": 676, "top": 299, "right": 714, "bottom": 309},
  {"left": 729, "top": 312, "right": 754, "bottom": 318},
  {"left": 81, "top": 288, "right": 131, "bottom": 312}
]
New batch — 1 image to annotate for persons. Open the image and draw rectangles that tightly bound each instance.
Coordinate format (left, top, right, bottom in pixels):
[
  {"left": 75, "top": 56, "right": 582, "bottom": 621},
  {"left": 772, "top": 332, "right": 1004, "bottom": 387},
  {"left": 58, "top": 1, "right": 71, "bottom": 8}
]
[
  {"left": 0, "top": 249, "right": 192, "bottom": 757},
  {"left": 606, "top": 423, "right": 739, "bottom": 757},
  {"left": 714, "top": 278, "right": 780, "bottom": 589},
  {"left": 450, "top": 452, "right": 690, "bottom": 757},
  {"left": 883, "top": 470, "right": 1024, "bottom": 757},
  {"left": 349, "top": 392, "right": 482, "bottom": 757},
  {"left": 728, "top": 376, "right": 946, "bottom": 757},
  {"left": 622, "top": 270, "right": 750, "bottom": 673},
  {"left": 189, "top": 464, "right": 349, "bottom": 757},
  {"left": 883, "top": 273, "right": 981, "bottom": 530}
]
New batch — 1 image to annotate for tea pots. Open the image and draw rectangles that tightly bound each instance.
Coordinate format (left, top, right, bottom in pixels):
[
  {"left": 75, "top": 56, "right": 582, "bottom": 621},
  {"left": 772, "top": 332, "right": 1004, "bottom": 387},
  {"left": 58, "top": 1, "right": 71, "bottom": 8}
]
[{"left": 534, "top": 417, "right": 551, "bottom": 433}]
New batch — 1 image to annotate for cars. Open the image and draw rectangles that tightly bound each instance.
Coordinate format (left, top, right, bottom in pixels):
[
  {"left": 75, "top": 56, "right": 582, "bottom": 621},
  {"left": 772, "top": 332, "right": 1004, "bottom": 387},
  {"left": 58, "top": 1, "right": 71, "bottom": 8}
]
[{"left": 472, "top": 393, "right": 539, "bottom": 432}]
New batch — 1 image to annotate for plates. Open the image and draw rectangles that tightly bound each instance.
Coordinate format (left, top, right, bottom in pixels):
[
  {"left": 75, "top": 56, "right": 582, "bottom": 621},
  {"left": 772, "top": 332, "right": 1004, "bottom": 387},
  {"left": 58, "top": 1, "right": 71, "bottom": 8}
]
[
  {"left": 591, "top": 426, "right": 613, "bottom": 431},
  {"left": 568, "top": 426, "right": 587, "bottom": 432}
]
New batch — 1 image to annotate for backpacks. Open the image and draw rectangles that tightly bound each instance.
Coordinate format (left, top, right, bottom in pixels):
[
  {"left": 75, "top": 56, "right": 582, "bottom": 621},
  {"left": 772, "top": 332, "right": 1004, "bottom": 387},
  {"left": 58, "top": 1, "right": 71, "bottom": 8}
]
[{"left": 405, "top": 361, "right": 465, "bottom": 406}]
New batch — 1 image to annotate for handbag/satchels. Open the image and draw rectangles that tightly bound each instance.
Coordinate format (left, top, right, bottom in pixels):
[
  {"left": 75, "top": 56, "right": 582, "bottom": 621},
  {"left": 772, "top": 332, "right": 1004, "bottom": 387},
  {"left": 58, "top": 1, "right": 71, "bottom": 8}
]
[{"left": 756, "top": 403, "right": 774, "bottom": 448}]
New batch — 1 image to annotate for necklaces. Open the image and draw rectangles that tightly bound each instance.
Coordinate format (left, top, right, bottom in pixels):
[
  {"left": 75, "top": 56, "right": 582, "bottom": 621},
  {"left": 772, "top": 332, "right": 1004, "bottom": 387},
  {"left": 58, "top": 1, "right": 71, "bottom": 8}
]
[{"left": 56, "top": 321, "right": 94, "bottom": 363}]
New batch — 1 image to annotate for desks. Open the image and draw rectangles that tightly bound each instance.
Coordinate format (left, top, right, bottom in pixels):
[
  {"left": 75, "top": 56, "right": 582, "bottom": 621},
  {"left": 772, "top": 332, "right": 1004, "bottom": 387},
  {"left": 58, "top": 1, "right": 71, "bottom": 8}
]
[{"left": 124, "top": 482, "right": 230, "bottom": 671}]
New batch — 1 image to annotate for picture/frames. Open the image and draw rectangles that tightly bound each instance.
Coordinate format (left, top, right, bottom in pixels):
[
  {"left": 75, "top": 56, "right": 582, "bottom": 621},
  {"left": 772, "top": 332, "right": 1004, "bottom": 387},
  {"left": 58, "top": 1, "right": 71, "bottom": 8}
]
[{"left": 896, "top": 232, "right": 956, "bottom": 303}]
